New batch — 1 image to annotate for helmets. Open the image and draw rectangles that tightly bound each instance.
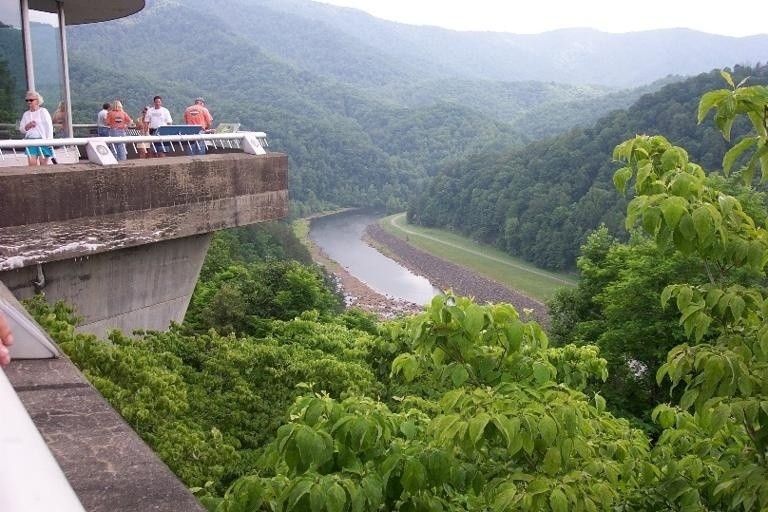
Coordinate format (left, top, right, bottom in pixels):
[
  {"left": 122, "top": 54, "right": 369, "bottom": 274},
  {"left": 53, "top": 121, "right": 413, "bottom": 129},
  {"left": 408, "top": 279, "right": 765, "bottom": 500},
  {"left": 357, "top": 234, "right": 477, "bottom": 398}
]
[{"left": 195, "top": 97, "right": 205, "bottom": 105}]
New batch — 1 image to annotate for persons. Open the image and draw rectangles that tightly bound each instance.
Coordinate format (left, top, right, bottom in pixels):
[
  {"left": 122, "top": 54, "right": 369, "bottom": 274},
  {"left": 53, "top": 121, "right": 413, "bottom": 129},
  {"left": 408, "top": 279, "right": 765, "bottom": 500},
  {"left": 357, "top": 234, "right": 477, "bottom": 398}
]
[
  {"left": 182, "top": 97, "right": 213, "bottom": 155},
  {"left": 135, "top": 106, "right": 150, "bottom": 159},
  {"left": 97, "top": 102, "right": 110, "bottom": 136},
  {"left": 143, "top": 96, "right": 173, "bottom": 153},
  {"left": 19, "top": 90, "right": 53, "bottom": 165},
  {"left": 104, "top": 100, "right": 132, "bottom": 160},
  {"left": 0, "top": 310, "right": 14, "bottom": 366},
  {"left": 50, "top": 99, "right": 65, "bottom": 137}
]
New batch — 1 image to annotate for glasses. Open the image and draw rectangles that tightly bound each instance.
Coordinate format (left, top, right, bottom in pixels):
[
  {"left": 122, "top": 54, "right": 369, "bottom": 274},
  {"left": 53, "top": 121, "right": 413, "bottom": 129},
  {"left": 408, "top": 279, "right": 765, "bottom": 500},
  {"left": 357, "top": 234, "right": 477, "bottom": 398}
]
[{"left": 25, "top": 99, "right": 36, "bottom": 102}]
